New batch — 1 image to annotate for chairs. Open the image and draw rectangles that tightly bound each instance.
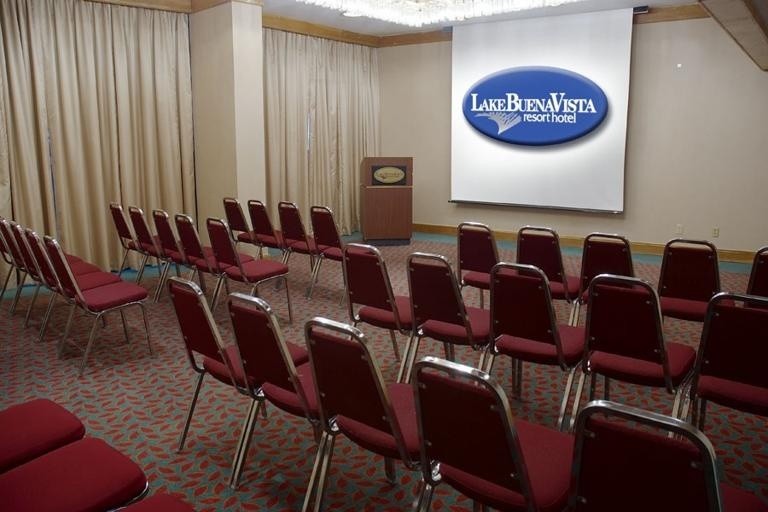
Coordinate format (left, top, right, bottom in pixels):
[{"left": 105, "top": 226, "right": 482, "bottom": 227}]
[
  {"left": 226, "top": 293, "right": 328, "bottom": 512},
  {"left": 0, "top": 195, "right": 767, "bottom": 512},
  {"left": 303, "top": 316, "right": 424, "bottom": 512},
  {"left": 404, "top": 252, "right": 516, "bottom": 401},
  {"left": 342, "top": 243, "right": 448, "bottom": 384},
  {"left": 128, "top": 197, "right": 295, "bottom": 329},
  {"left": 1, "top": 202, "right": 156, "bottom": 377},
  {"left": 1, "top": 437, "right": 148, "bottom": 512},
  {"left": 166, "top": 277, "right": 267, "bottom": 485},
  {"left": 116, "top": 490, "right": 194, "bottom": 512},
  {"left": 273, "top": 201, "right": 348, "bottom": 309},
  {"left": 458, "top": 221, "right": 766, "bottom": 447},
  {"left": 0, "top": 399, "right": 86, "bottom": 472},
  {"left": 563, "top": 399, "right": 768, "bottom": 512},
  {"left": 413, "top": 355, "right": 574, "bottom": 510}
]
